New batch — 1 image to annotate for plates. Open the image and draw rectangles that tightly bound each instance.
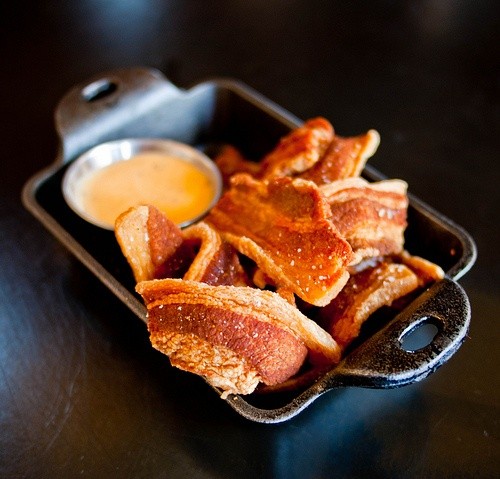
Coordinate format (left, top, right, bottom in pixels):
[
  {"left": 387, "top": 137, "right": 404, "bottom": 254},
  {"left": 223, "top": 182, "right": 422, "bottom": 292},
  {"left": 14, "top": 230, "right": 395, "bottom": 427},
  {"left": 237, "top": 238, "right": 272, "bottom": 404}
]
[{"left": 21, "top": 66, "right": 478, "bottom": 423}]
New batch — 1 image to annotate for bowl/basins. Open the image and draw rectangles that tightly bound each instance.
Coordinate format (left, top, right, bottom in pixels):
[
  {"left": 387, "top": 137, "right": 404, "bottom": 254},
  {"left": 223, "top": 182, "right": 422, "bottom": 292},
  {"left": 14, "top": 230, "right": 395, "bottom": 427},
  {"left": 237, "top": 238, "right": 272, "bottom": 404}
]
[{"left": 62, "top": 137, "right": 222, "bottom": 232}]
